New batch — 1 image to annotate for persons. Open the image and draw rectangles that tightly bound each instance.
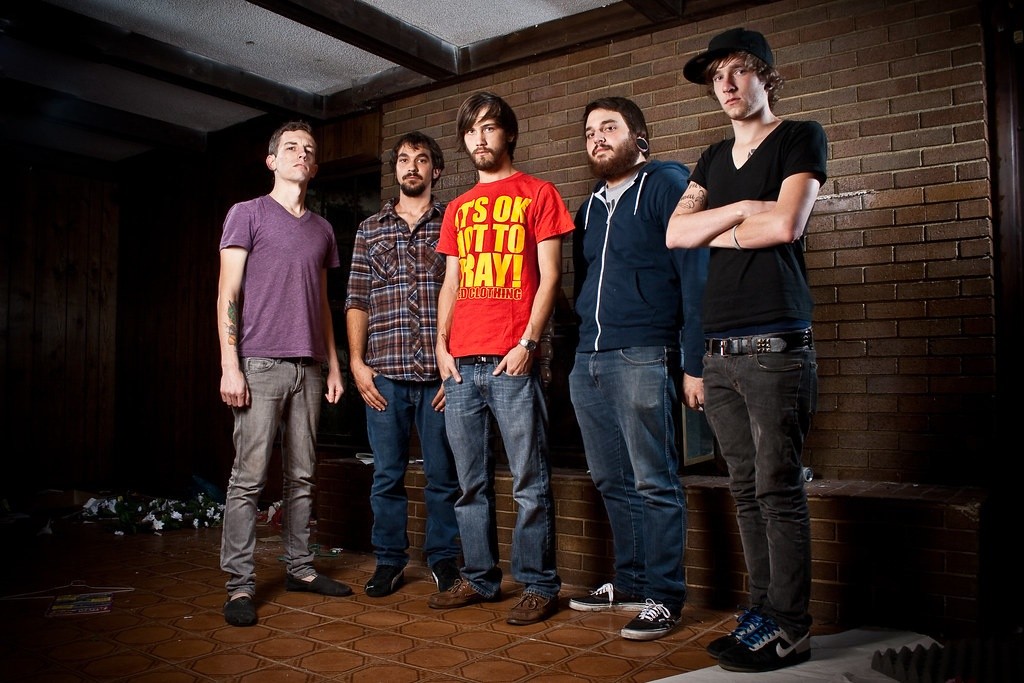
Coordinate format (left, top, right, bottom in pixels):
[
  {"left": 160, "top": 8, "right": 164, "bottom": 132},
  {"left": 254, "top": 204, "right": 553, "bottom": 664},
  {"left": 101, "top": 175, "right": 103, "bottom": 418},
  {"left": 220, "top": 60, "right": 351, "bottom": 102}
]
[
  {"left": 217, "top": 122, "right": 351, "bottom": 626},
  {"left": 569, "top": 95, "right": 709, "bottom": 640},
  {"left": 345, "top": 130, "right": 464, "bottom": 597},
  {"left": 427, "top": 91, "right": 574, "bottom": 626},
  {"left": 666, "top": 28, "right": 827, "bottom": 674}
]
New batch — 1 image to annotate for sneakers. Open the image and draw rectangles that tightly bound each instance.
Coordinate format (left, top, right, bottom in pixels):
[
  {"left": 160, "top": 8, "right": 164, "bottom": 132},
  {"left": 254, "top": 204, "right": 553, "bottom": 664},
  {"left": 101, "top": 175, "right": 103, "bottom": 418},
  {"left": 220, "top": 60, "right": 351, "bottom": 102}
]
[
  {"left": 286, "top": 572, "right": 352, "bottom": 597},
  {"left": 430, "top": 557, "right": 460, "bottom": 593},
  {"left": 620, "top": 603, "right": 683, "bottom": 641},
  {"left": 506, "top": 592, "right": 560, "bottom": 625},
  {"left": 569, "top": 582, "right": 649, "bottom": 611},
  {"left": 365, "top": 563, "right": 407, "bottom": 596},
  {"left": 707, "top": 607, "right": 813, "bottom": 672},
  {"left": 428, "top": 581, "right": 503, "bottom": 610},
  {"left": 226, "top": 590, "right": 259, "bottom": 628}
]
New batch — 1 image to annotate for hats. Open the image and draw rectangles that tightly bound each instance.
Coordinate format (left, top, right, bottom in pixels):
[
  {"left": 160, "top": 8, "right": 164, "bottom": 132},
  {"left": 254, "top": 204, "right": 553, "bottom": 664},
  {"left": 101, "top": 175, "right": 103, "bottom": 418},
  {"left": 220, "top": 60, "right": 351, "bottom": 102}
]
[{"left": 682, "top": 28, "right": 774, "bottom": 85}]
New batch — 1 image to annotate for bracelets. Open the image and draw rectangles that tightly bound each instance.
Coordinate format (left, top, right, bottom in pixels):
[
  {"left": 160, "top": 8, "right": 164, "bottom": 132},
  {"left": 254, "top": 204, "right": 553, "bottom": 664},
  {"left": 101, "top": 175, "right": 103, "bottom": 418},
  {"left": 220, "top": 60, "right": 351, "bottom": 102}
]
[{"left": 732, "top": 224, "right": 742, "bottom": 250}]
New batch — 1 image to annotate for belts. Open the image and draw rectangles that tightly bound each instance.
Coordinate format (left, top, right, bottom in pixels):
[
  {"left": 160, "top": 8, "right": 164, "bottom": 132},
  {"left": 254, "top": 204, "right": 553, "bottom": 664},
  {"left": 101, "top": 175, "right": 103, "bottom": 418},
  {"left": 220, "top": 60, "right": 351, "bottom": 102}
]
[
  {"left": 458, "top": 354, "right": 500, "bottom": 365},
  {"left": 282, "top": 356, "right": 313, "bottom": 366},
  {"left": 706, "top": 330, "right": 813, "bottom": 356}
]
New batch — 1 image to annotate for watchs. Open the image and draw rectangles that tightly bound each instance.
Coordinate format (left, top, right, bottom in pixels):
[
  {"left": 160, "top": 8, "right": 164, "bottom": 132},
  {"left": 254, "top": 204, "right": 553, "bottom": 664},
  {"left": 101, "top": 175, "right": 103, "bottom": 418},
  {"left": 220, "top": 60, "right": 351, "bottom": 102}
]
[{"left": 519, "top": 338, "right": 536, "bottom": 352}]
